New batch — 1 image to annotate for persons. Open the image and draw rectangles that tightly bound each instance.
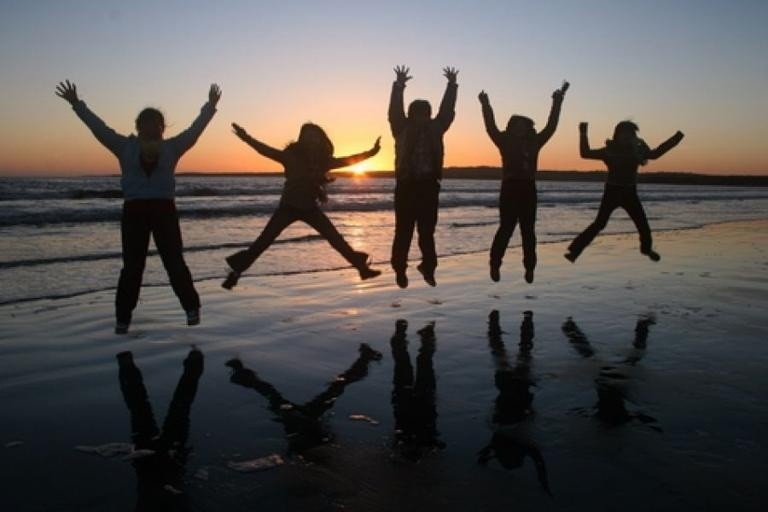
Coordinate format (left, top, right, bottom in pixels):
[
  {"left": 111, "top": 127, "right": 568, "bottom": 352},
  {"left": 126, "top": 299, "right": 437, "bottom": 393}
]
[
  {"left": 476, "top": 306, "right": 554, "bottom": 498},
  {"left": 558, "top": 309, "right": 660, "bottom": 430},
  {"left": 391, "top": 317, "right": 465, "bottom": 494},
  {"left": 564, "top": 120, "right": 685, "bottom": 263},
  {"left": 475, "top": 80, "right": 570, "bottom": 283},
  {"left": 222, "top": 343, "right": 383, "bottom": 468},
  {"left": 221, "top": 122, "right": 382, "bottom": 290},
  {"left": 75, "top": 347, "right": 206, "bottom": 512},
  {"left": 53, "top": 78, "right": 223, "bottom": 335},
  {"left": 390, "top": 64, "right": 460, "bottom": 289}
]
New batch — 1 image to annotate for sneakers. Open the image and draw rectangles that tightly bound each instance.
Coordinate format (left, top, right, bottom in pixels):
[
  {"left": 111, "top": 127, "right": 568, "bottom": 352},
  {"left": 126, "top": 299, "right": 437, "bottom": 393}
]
[
  {"left": 490, "top": 259, "right": 500, "bottom": 281},
  {"left": 524, "top": 262, "right": 534, "bottom": 283},
  {"left": 182, "top": 349, "right": 204, "bottom": 370},
  {"left": 395, "top": 265, "right": 408, "bottom": 288},
  {"left": 565, "top": 254, "right": 576, "bottom": 262},
  {"left": 358, "top": 268, "right": 381, "bottom": 280},
  {"left": 394, "top": 320, "right": 408, "bottom": 339},
  {"left": 225, "top": 358, "right": 245, "bottom": 371},
  {"left": 222, "top": 271, "right": 237, "bottom": 290},
  {"left": 187, "top": 312, "right": 200, "bottom": 326},
  {"left": 417, "top": 262, "right": 437, "bottom": 287},
  {"left": 117, "top": 350, "right": 133, "bottom": 363},
  {"left": 359, "top": 343, "right": 381, "bottom": 361},
  {"left": 115, "top": 323, "right": 130, "bottom": 335},
  {"left": 416, "top": 323, "right": 435, "bottom": 336},
  {"left": 642, "top": 250, "right": 660, "bottom": 261}
]
